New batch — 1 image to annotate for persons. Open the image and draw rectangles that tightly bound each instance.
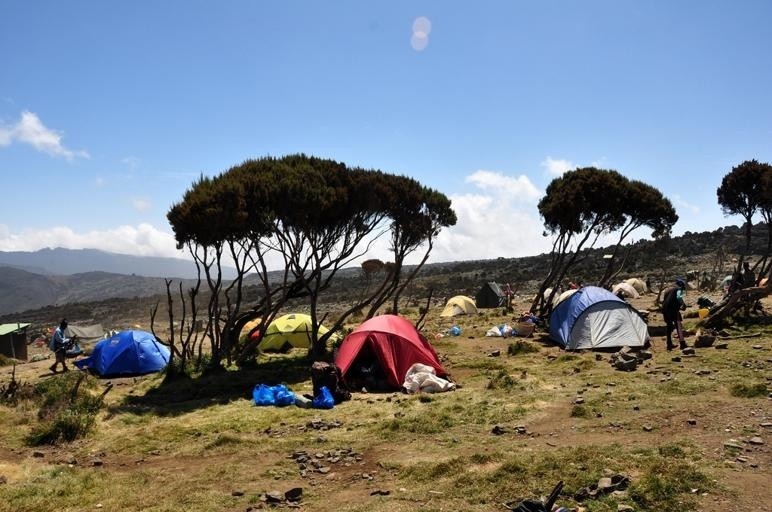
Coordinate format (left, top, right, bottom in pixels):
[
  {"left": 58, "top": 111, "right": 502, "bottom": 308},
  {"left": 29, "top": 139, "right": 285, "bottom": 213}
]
[
  {"left": 48, "top": 317, "right": 72, "bottom": 374},
  {"left": 663, "top": 278, "right": 694, "bottom": 351},
  {"left": 75, "top": 342, "right": 80, "bottom": 360},
  {"left": 112, "top": 328, "right": 116, "bottom": 337},
  {"left": 646, "top": 278, "right": 654, "bottom": 293},
  {"left": 743, "top": 261, "right": 755, "bottom": 288},
  {"left": 616, "top": 290, "right": 625, "bottom": 301}
]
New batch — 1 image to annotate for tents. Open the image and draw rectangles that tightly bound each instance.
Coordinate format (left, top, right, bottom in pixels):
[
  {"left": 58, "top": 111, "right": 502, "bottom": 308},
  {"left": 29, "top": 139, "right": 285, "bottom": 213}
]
[
  {"left": 74, "top": 328, "right": 173, "bottom": 378},
  {"left": 625, "top": 278, "right": 648, "bottom": 295},
  {"left": 611, "top": 283, "right": 640, "bottom": 299},
  {"left": 256, "top": 311, "right": 342, "bottom": 351},
  {"left": 233, "top": 316, "right": 263, "bottom": 351},
  {"left": 334, "top": 313, "right": 451, "bottom": 392},
  {"left": 439, "top": 294, "right": 480, "bottom": 317},
  {"left": 717, "top": 275, "right": 733, "bottom": 289},
  {"left": 549, "top": 283, "right": 654, "bottom": 353}
]
